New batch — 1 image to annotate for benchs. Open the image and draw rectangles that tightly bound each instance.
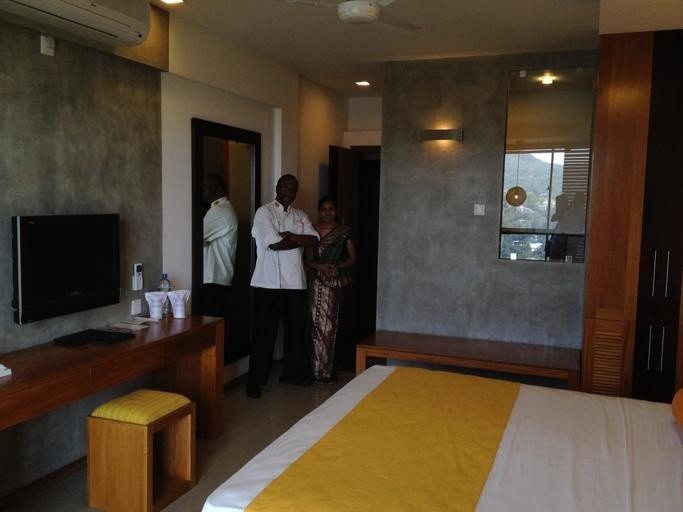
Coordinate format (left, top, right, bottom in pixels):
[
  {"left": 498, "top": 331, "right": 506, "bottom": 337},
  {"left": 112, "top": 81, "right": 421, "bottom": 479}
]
[{"left": 355, "top": 330, "right": 580, "bottom": 393}]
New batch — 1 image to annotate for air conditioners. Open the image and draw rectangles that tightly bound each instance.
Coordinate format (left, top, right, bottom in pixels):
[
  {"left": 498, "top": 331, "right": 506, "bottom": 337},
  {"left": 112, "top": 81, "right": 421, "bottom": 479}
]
[{"left": 0, "top": 0, "right": 150, "bottom": 55}]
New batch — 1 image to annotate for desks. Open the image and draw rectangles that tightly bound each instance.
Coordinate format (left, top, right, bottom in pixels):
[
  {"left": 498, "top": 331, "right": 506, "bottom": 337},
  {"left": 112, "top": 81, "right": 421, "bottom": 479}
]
[{"left": 0, "top": 313, "right": 224, "bottom": 441}]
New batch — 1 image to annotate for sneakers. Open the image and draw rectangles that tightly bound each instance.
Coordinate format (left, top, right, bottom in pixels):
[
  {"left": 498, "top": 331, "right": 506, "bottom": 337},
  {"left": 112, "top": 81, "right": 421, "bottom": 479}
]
[
  {"left": 279, "top": 376, "right": 312, "bottom": 387},
  {"left": 246, "top": 385, "right": 262, "bottom": 399}
]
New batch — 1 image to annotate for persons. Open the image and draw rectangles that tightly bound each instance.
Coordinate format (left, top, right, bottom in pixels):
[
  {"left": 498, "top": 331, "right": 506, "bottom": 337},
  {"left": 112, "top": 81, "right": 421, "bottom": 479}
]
[
  {"left": 551, "top": 190, "right": 585, "bottom": 233},
  {"left": 302, "top": 195, "right": 359, "bottom": 386},
  {"left": 245, "top": 174, "right": 322, "bottom": 398},
  {"left": 203, "top": 174, "right": 240, "bottom": 316}
]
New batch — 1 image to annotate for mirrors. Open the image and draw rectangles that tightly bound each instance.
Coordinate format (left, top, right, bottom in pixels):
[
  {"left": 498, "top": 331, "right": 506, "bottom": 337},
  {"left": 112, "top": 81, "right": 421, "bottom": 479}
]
[
  {"left": 496, "top": 66, "right": 596, "bottom": 263},
  {"left": 191, "top": 117, "right": 260, "bottom": 366}
]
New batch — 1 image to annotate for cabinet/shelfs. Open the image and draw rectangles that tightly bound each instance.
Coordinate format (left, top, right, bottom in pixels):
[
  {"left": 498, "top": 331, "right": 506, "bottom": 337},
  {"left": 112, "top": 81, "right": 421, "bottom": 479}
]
[{"left": 631, "top": 28, "right": 682, "bottom": 407}]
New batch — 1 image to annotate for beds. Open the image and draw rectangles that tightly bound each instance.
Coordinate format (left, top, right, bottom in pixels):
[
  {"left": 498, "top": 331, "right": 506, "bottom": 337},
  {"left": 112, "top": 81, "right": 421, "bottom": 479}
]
[{"left": 200, "top": 364, "right": 682, "bottom": 512}]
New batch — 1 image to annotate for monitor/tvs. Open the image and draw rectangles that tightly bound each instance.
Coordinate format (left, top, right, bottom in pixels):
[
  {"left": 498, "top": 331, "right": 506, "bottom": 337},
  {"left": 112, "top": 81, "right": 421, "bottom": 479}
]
[{"left": 11, "top": 213, "right": 120, "bottom": 325}]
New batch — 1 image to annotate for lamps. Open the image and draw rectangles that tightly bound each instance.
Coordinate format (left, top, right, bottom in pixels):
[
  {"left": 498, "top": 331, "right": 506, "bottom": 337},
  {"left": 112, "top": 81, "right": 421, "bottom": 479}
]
[{"left": 505, "top": 154, "right": 525, "bottom": 206}]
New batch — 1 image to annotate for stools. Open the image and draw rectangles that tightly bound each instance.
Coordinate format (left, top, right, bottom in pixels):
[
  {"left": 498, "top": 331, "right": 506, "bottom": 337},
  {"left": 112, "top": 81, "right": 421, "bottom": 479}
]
[{"left": 85, "top": 389, "right": 196, "bottom": 512}]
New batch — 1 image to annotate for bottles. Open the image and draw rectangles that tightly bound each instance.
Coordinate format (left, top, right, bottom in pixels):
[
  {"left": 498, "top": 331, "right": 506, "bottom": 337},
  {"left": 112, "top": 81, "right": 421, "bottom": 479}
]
[{"left": 158, "top": 273, "right": 170, "bottom": 315}]
